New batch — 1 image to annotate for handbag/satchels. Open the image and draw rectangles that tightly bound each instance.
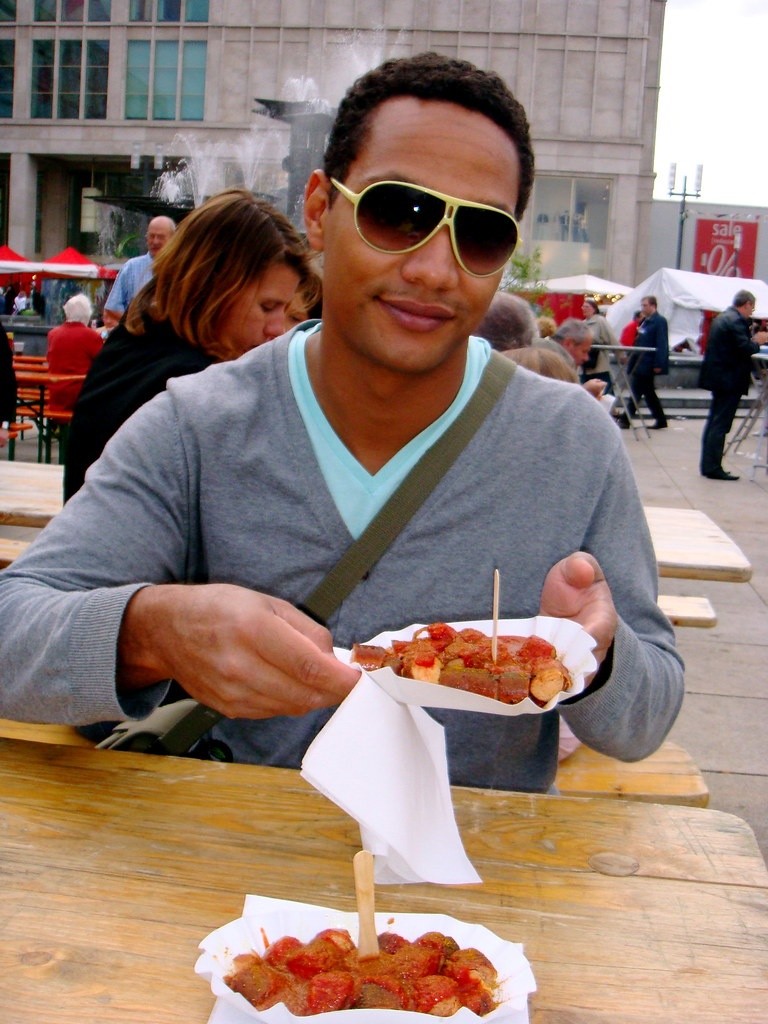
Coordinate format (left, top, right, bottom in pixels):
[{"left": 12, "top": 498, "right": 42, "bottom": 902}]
[
  {"left": 696, "top": 359, "right": 714, "bottom": 388},
  {"left": 94, "top": 698, "right": 233, "bottom": 764}
]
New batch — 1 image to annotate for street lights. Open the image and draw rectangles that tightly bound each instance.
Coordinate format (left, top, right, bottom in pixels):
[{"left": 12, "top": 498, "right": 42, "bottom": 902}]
[
  {"left": 130, "top": 142, "right": 165, "bottom": 255},
  {"left": 668, "top": 162, "right": 703, "bottom": 270}
]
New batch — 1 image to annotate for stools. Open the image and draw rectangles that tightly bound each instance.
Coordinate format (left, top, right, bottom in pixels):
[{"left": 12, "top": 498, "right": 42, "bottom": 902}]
[{"left": 8, "top": 422, "right": 34, "bottom": 460}]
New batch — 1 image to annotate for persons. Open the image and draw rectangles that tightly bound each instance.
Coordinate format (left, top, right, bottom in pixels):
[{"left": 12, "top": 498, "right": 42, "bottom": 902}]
[
  {"left": 696, "top": 291, "right": 768, "bottom": 481},
  {"left": 0, "top": 322, "right": 18, "bottom": 421},
  {"left": 46, "top": 189, "right": 667, "bottom": 505},
  {"left": 0, "top": 287, "right": 46, "bottom": 319},
  {"left": 0, "top": 50, "right": 687, "bottom": 795}
]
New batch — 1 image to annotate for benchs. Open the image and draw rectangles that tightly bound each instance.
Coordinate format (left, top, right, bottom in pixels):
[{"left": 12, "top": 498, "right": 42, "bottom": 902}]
[
  {"left": 1, "top": 537, "right": 721, "bottom": 630},
  {"left": 1, "top": 720, "right": 712, "bottom": 808}
]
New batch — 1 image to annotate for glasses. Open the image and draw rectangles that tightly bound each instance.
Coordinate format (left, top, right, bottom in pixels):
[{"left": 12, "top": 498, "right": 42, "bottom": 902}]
[
  {"left": 330, "top": 175, "right": 523, "bottom": 278},
  {"left": 752, "top": 307, "right": 755, "bottom": 312}
]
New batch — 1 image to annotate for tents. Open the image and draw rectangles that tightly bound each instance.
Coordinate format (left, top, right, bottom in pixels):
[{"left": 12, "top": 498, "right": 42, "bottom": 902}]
[
  {"left": 507, "top": 267, "right": 768, "bottom": 351},
  {"left": 0, "top": 245, "right": 118, "bottom": 294}
]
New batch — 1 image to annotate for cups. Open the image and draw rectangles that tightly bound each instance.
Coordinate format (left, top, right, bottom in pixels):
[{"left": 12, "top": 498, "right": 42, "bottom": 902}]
[{"left": 14, "top": 342, "right": 25, "bottom": 356}]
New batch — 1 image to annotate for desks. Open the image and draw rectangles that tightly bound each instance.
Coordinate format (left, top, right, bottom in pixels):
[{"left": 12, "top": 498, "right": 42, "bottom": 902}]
[
  {"left": 13, "top": 354, "right": 87, "bottom": 463},
  {"left": 0, "top": 737, "right": 768, "bottom": 1024},
  {"left": 590, "top": 344, "right": 656, "bottom": 441},
  {"left": 723, "top": 353, "right": 768, "bottom": 482},
  {"left": 0, "top": 459, "right": 753, "bottom": 586}
]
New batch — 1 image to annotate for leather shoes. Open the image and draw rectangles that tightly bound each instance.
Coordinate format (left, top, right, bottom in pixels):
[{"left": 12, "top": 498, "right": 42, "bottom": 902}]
[
  {"left": 702, "top": 471, "right": 739, "bottom": 480},
  {"left": 646, "top": 424, "right": 667, "bottom": 429}
]
